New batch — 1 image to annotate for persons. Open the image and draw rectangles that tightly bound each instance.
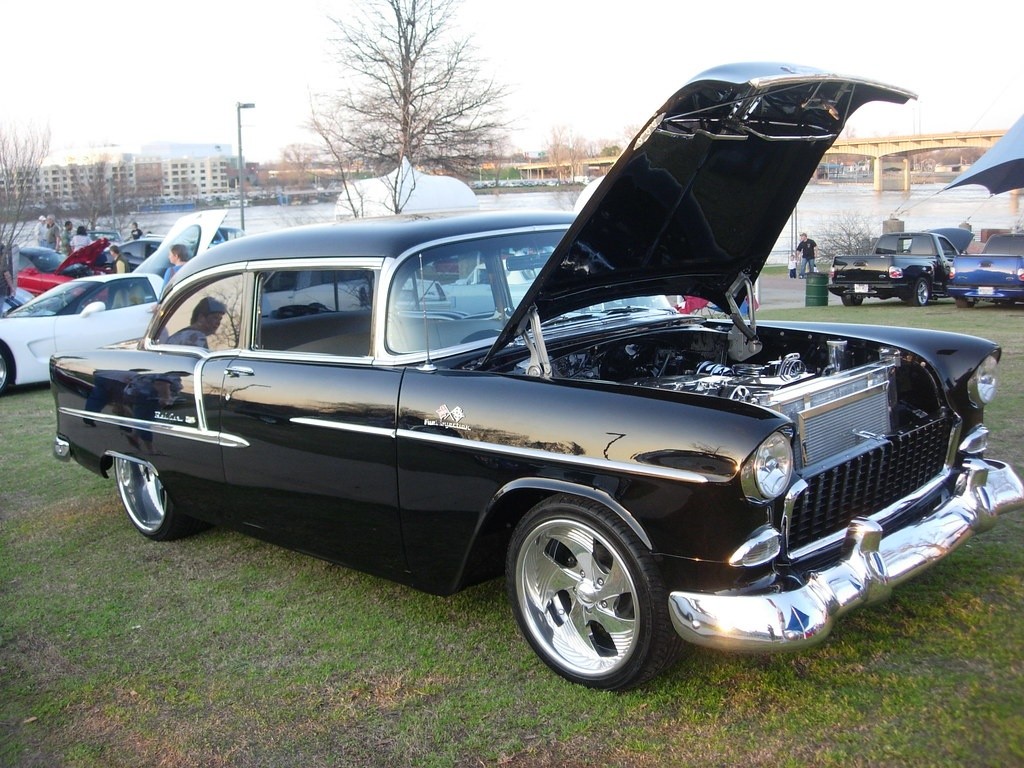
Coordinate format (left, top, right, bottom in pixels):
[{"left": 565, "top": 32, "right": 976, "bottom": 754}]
[
  {"left": 788, "top": 255, "right": 799, "bottom": 279},
  {"left": 35, "top": 213, "right": 142, "bottom": 275},
  {"left": 795, "top": 232, "right": 818, "bottom": 278},
  {"left": 0, "top": 243, "right": 17, "bottom": 316},
  {"left": 164, "top": 244, "right": 192, "bottom": 287},
  {"left": 82, "top": 296, "right": 228, "bottom": 452}
]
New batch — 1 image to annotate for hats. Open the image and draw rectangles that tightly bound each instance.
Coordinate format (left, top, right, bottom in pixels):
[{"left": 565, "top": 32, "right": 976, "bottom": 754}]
[
  {"left": 38, "top": 215, "right": 47, "bottom": 221},
  {"left": 799, "top": 233, "right": 807, "bottom": 239}
]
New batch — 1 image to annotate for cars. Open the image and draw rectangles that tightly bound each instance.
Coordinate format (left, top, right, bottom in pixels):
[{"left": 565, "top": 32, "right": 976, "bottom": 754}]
[
  {"left": 0, "top": 208, "right": 368, "bottom": 397},
  {"left": 46, "top": 59, "right": 1024, "bottom": 670}
]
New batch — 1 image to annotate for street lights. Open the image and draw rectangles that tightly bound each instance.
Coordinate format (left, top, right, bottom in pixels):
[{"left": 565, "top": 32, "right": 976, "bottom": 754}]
[{"left": 235, "top": 100, "right": 256, "bottom": 230}]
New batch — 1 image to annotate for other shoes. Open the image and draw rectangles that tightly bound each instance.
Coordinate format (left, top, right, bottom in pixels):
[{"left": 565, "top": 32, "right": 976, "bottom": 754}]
[{"left": 800, "top": 276, "right": 803, "bottom": 280}]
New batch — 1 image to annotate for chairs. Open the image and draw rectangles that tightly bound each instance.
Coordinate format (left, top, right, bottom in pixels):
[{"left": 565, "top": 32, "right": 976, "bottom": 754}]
[{"left": 111, "top": 286, "right": 145, "bottom": 309}]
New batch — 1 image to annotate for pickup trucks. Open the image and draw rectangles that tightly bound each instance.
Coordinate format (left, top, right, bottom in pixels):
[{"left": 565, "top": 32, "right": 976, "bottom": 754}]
[
  {"left": 826, "top": 228, "right": 975, "bottom": 307},
  {"left": 944, "top": 231, "right": 1024, "bottom": 306}
]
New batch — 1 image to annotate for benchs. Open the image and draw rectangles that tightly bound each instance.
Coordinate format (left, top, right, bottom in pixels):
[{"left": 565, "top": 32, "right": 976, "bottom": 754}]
[
  {"left": 261, "top": 310, "right": 371, "bottom": 350},
  {"left": 286, "top": 320, "right": 504, "bottom": 357}
]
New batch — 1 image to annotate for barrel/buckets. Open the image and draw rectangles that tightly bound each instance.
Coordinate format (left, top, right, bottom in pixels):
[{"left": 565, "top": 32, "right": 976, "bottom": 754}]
[{"left": 804, "top": 272, "right": 829, "bottom": 307}]
